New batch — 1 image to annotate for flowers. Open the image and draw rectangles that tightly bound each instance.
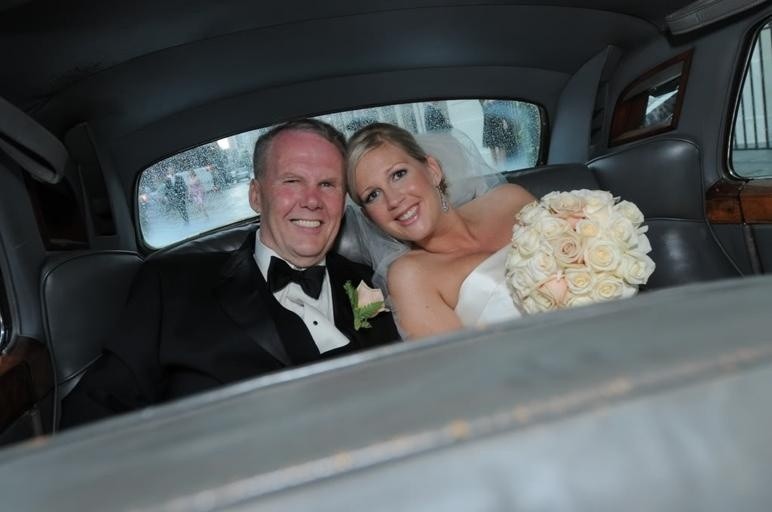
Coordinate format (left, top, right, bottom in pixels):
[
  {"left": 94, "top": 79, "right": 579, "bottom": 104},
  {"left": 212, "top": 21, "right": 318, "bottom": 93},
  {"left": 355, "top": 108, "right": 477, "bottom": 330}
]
[
  {"left": 505, "top": 188, "right": 656, "bottom": 316},
  {"left": 344, "top": 278, "right": 391, "bottom": 333}
]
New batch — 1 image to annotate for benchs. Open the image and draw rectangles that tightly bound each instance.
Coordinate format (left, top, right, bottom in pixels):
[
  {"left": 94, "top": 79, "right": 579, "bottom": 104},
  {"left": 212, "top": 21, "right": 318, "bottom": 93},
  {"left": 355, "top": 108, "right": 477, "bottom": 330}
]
[{"left": 40, "top": 136, "right": 748, "bottom": 437}]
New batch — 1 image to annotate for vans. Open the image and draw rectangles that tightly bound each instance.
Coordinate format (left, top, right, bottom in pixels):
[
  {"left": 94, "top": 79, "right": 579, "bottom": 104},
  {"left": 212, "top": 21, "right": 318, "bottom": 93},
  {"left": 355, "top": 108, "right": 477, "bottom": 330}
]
[{"left": 158, "top": 165, "right": 223, "bottom": 195}]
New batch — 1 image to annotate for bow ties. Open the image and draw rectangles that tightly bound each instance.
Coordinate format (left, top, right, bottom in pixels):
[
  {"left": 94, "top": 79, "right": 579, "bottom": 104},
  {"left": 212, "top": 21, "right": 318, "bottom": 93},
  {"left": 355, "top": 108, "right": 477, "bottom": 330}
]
[{"left": 265, "top": 255, "right": 327, "bottom": 301}]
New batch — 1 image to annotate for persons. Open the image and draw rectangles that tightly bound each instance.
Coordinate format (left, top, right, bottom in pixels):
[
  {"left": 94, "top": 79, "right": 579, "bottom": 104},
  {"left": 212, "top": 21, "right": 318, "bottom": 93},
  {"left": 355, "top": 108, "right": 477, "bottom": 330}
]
[
  {"left": 105, "top": 118, "right": 401, "bottom": 414},
  {"left": 162, "top": 166, "right": 189, "bottom": 220},
  {"left": 345, "top": 123, "right": 539, "bottom": 339},
  {"left": 186, "top": 170, "right": 209, "bottom": 220}
]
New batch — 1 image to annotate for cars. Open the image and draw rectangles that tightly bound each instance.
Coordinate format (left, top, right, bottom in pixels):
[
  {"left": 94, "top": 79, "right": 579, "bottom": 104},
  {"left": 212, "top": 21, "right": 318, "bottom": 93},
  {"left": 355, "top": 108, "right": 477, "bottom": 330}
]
[{"left": 236, "top": 169, "right": 250, "bottom": 181}]
